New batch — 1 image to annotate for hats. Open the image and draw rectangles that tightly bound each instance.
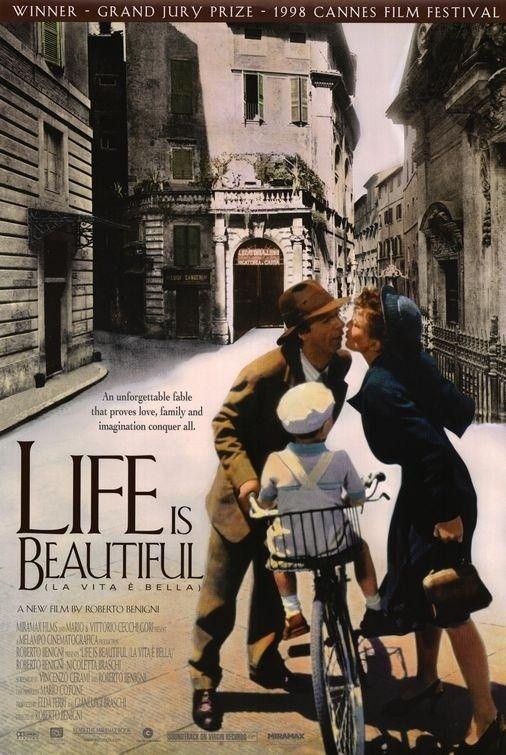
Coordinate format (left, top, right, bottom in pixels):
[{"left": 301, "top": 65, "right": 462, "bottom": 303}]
[
  {"left": 276, "top": 380, "right": 336, "bottom": 434},
  {"left": 275, "top": 279, "right": 351, "bottom": 346},
  {"left": 379, "top": 284, "right": 423, "bottom": 361}
]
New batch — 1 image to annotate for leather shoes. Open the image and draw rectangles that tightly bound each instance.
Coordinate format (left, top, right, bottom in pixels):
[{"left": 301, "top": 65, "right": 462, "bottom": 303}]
[
  {"left": 249, "top": 657, "right": 298, "bottom": 693},
  {"left": 192, "top": 688, "right": 223, "bottom": 731}
]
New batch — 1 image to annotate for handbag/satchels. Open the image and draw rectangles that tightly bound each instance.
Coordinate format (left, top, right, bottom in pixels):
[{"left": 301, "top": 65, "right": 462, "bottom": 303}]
[{"left": 420, "top": 559, "right": 495, "bottom": 626}]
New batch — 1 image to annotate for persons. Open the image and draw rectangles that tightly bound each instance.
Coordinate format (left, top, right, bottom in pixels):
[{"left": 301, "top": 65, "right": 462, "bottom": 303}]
[
  {"left": 256, "top": 382, "right": 401, "bottom": 639},
  {"left": 187, "top": 280, "right": 353, "bottom": 731},
  {"left": 341, "top": 282, "right": 506, "bottom": 755}
]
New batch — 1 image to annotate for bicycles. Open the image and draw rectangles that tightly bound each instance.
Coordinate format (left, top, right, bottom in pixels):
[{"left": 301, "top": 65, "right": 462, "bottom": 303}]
[{"left": 247, "top": 471, "right": 390, "bottom": 754}]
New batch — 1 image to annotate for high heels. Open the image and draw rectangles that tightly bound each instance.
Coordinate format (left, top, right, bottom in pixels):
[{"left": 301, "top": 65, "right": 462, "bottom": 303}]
[{"left": 381, "top": 676, "right": 505, "bottom": 754}]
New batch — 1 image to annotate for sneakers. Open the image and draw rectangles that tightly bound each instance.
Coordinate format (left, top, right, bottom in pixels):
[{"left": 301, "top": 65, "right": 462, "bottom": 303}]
[
  {"left": 282, "top": 613, "right": 310, "bottom": 641},
  {"left": 360, "top": 608, "right": 386, "bottom": 633}
]
